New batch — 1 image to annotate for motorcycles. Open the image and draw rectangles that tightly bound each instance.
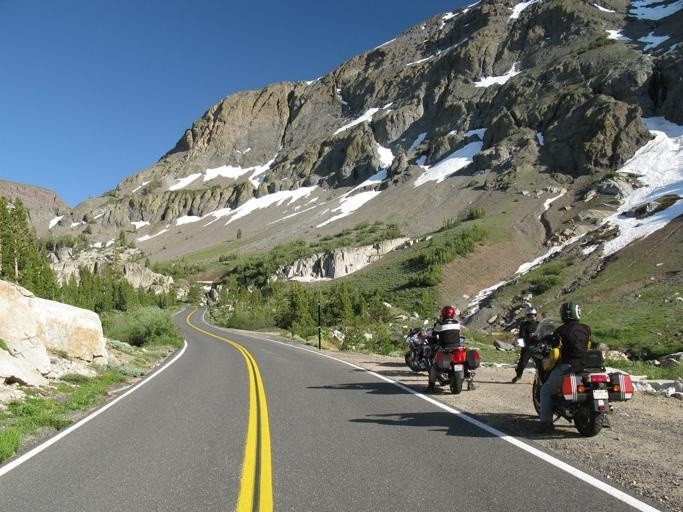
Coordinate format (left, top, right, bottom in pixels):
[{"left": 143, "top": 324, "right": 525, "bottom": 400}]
[
  {"left": 526, "top": 315, "right": 633, "bottom": 437},
  {"left": 420, "top": 331, "right": 480, "bottom": 394},
  {"left": 401, "top": 319, "right": 432, "bottom": 372}
]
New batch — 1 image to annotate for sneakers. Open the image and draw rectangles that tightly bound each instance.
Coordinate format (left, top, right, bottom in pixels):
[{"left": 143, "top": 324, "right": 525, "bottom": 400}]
[{"left": 512, "top": 376, "right": 521, "bottom": 383}]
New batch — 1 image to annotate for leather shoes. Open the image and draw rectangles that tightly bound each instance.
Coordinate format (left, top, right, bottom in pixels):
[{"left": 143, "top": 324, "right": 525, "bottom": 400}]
[
  {"left": 601, "top": 415, "right": 611, "bottom": 427},
  {"left": 532, "top": 422, "right": 554, "bottom": 434}
]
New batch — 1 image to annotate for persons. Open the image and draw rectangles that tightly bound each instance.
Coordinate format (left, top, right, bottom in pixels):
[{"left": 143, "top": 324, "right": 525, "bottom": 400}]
[
  {"left": 421, "top": 306, "right": 476, "bottom": 391},
  {"left": 510, "top": 307, "right": 544, "bottom": 384},
  {"left": 535, "top": 301, "right": 607, "bottom": 435}
]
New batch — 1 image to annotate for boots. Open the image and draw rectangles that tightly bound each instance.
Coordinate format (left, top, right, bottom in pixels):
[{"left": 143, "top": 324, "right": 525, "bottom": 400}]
[
  {"left": 423, "top": 384, "right": 434, "bottom": 392},
  {"left": 468, "top": 381, "right": 476, "bottom": 390}
]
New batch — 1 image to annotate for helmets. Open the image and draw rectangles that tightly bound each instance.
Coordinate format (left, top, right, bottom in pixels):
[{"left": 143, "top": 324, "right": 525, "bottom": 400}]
[
  {"left": 559, "top": 302, "right": 580, "bottom": 322},
  {"left": 441, "top": 306, "right": 454, "bottom": 319},
  {"left": 524, "top": 307, "right": 536, "bottom": 322}
]
